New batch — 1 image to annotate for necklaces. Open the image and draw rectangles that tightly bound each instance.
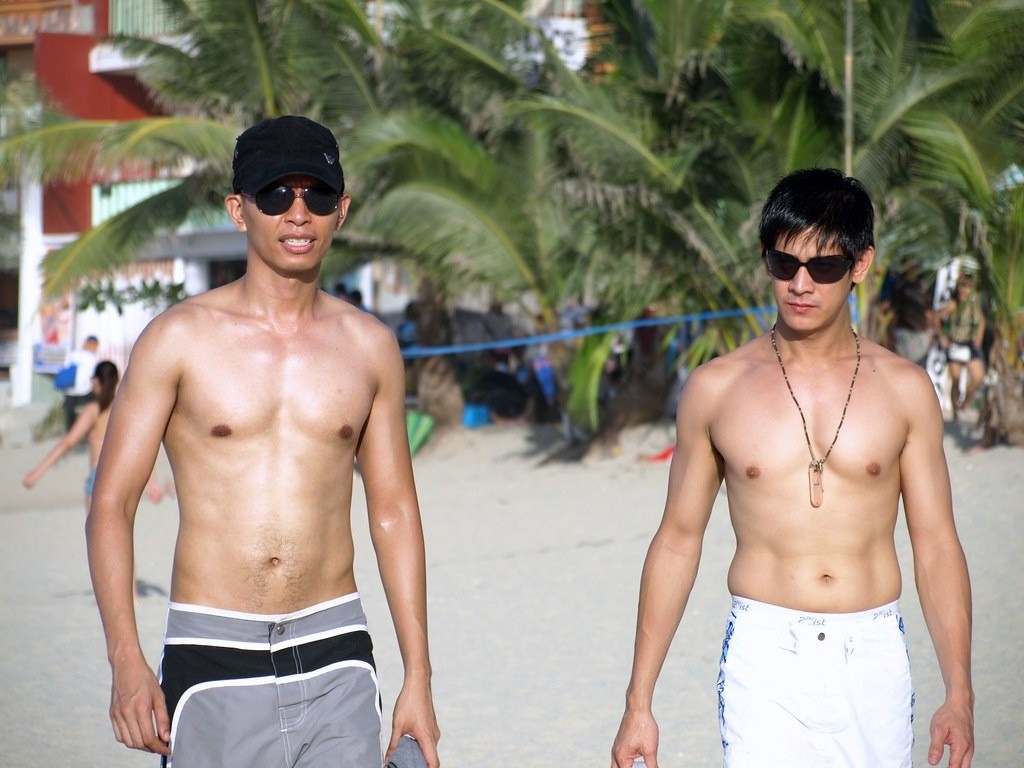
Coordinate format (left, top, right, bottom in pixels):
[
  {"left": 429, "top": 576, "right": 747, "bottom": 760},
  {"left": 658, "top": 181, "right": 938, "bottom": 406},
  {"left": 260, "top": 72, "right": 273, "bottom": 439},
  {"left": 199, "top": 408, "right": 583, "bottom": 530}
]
[{"left": 771, "top": 322, "right": 862, "bottom": 507}]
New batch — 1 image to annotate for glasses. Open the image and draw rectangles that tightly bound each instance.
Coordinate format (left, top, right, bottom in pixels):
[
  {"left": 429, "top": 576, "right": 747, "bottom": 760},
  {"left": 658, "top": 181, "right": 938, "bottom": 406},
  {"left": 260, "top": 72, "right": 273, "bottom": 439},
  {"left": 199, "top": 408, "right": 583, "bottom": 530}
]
[
  {"left": 241, "top": 182, "right": 341, "bottom": 216},
  {"left": 762, "top": 248, "right": 852, "bottom": 284}
]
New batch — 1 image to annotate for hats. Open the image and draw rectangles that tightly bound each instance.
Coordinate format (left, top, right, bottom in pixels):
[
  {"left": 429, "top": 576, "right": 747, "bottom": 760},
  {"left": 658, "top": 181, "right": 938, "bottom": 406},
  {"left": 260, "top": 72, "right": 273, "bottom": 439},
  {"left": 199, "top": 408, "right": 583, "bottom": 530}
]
[{"left": 232, "top": 115, "right": 343, "bottom": 197}]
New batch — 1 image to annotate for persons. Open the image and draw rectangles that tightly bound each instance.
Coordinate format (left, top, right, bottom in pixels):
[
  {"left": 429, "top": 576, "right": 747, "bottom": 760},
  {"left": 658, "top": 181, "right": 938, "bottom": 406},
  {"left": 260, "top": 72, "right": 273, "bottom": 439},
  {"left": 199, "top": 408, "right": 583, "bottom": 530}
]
[
  {"left": 928, "top": 269, "right": 990, "bottom": 424},
  {"left": 607, "top": 165, "right": 976, "bottom": 768},
  {"left": 85, "top": 114, "right": 449, "bottom": 768},
  {"left": 21, "top": 286, "right": 692, "bottom": 508}
]
